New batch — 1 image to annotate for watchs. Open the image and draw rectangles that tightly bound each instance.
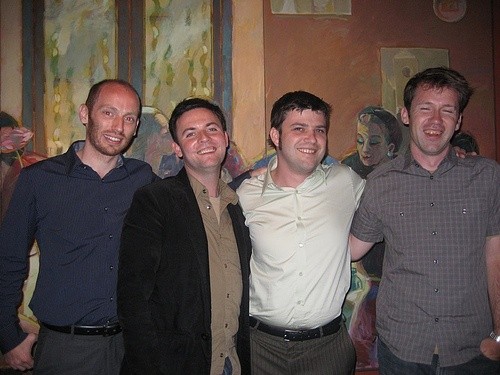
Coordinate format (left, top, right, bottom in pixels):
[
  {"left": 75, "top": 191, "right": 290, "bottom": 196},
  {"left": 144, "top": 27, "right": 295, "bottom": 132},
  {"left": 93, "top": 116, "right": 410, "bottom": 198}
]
[{"left": 489, "top": 330, "right": 499, "bottom": 342}]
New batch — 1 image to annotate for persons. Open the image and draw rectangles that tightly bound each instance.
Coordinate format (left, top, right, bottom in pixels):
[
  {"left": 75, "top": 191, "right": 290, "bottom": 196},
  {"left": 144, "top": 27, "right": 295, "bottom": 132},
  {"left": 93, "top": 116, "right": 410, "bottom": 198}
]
[
  {"left": 1, "top": 78, "right": 268, "bottom": 374},
  {"left": 236, "top": 87, "right": 369, "bottom": 375},
  {"left": 348, "top": 68, "right": 500, "bottom": 375},
  {"left": 116, "top": 95, "right": 253, "bottom": 374}
]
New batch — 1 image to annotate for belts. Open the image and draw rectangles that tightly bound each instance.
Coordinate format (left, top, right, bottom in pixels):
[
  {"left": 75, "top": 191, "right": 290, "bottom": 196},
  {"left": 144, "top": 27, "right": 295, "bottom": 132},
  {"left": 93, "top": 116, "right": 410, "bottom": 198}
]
[
  {"left": 43, "top": 322, "right": 122, "bottom": 337},
  {"left": 248, "top": 317, "right": 342, "bottom": 341}
]
